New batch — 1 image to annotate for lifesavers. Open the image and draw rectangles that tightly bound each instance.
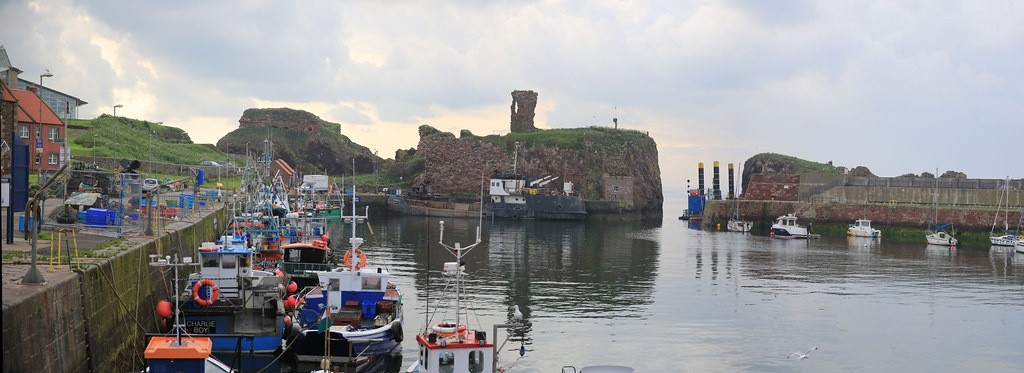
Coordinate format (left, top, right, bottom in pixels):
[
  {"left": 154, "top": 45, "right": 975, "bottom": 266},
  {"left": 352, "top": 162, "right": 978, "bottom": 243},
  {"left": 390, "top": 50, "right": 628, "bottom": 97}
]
[
  {"left": 312, "top": 240, "right": 328, "bottom": 248},
  {"left": 343, "top": 247, "right": 367, "bottom": 270},
  {"left": 192, "top": 277, "right": 220, "bottom": 305},
  {"left": 197, "top": 246, "right": 219, "bottom": 253},
  {"left": 432, "top": 320, "right": 467, "bottom": 339}
]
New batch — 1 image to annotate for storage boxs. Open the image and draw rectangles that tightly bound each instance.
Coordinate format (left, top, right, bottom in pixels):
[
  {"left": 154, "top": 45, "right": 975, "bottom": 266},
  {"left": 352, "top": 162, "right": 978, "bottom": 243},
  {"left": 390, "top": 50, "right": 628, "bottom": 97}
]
[
  {"left": 307, "top": 294, "right": 326, "bottom": 313},
  {"left": 19, "top": 215, "right": 41, "bottom": 232},
  {"left": 126, "top": 194, "right": 195, "bottom": 220},
  {"left": 199, "top": 201, "right": 205, "bottom": 206},
  {"left": 79, "top": 208, "right": 118, "bottom": 228},
  {"left": 192, "top": 282, "right": 210, "bottom": 299},
  {"left": 363, "top": 300, "right": 377, "bottom": 320},
  {"left": 206, "top": 191, "right": 216, "bottom": 201}
]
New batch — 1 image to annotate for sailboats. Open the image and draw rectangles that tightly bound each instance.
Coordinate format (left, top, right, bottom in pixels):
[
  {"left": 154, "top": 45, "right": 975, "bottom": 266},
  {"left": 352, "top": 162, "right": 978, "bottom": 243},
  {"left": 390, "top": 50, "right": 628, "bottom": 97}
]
[
  {"left": 726, "top": 161, "right": 754, "bottom": 233},
  {"left": 925, "top": 166, "right": 959, "bottom": 246},
  {"left": 988, "top": 174, "right": 1018, "bottom": 246}
]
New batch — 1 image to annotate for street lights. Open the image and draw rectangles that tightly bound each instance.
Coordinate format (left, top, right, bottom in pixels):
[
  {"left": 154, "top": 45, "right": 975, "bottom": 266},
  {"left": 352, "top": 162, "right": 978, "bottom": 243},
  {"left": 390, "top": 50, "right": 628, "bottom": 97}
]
[
  {"left": 39, "top": 68, "right": 54, "bottom": 186},
  {"left": 113, "top": 104, "right": 124, "bottom": 173},
  {"left": 149, "top": 121, "right": 163, "bottom": 176}
]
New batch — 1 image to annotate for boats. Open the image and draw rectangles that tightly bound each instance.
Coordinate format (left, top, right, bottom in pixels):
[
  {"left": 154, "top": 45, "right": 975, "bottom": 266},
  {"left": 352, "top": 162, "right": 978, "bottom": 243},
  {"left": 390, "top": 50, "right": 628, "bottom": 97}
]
[
  {"left": 770, "top": 212, "right": 822, "bottom": 239},
  {"left": 1014, "top": 233, "right": 1024, "bottom": 253},
  {"left": 846, "top": 217, "right": 882, "bottom": 239},
  {"left": 137, "top": 190, "right": 497, "bottom": 373}
]
